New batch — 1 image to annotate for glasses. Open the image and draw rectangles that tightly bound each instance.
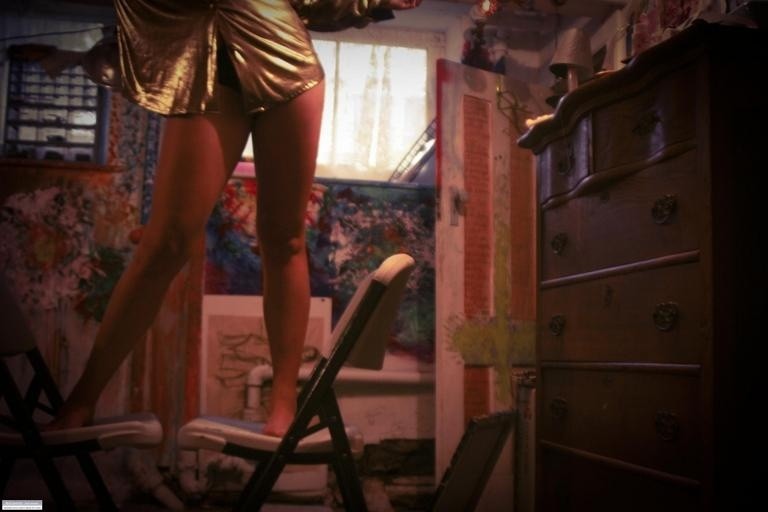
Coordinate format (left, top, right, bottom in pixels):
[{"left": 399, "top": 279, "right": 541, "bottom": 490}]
[{"left": 547, "top": 27, "right": 593, "bottom": 94}]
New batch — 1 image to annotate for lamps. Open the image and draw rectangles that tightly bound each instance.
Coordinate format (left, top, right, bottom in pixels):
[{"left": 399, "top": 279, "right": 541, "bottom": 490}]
[
  {"left": 0, "top": 284, "right": 166, "bottom": 511},
  {"left": 174, "top": 252, "right": 416, "bottom": 512}
]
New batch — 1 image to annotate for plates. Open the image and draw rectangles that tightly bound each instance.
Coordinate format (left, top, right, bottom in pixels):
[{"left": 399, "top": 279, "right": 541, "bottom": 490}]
[{"left": 513, "top": 16, "right": 765, "bottom": 511}]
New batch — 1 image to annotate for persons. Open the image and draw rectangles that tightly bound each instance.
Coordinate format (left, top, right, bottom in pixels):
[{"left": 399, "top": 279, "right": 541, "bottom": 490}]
[{"left": 43, "top": 0, "right": 423, "bottom": 438}]
